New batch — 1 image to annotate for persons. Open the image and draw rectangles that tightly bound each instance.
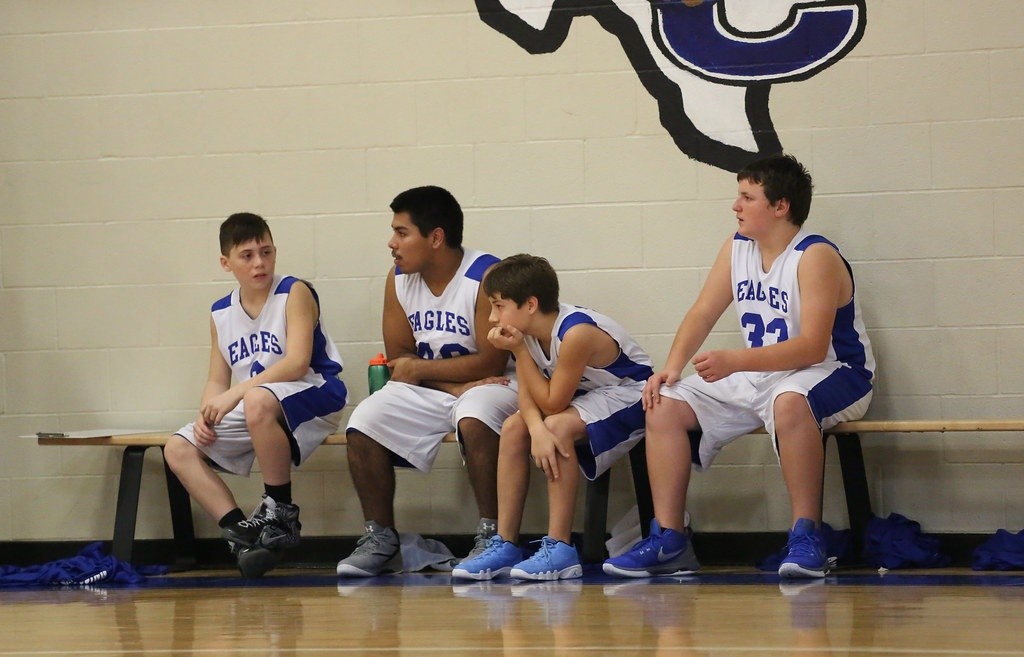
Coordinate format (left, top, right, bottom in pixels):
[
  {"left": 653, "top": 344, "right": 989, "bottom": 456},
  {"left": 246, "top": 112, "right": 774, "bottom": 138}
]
[
  {"left": 336, "top": 186, "right": 518, "bottom": 575},
  {"left": 451, "top": 253, "right": 656, "bottom": 580},
  {"left": 163, "top": 213, "right": 348, "bottom": 577},
  {"left": 603, "top": 156, "right": 876, "bottom": 579}
]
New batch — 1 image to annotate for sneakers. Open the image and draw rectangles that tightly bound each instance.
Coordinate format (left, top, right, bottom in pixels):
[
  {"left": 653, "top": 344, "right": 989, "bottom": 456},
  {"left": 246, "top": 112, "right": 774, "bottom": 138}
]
[
  {"left": 221, "top": 493, "right": 303, "bottom": 551},
  {"left": 226, "top": 541, "right": 284, "bottom": 581},
  {"left": 509, "top": 535, "right": 583, "bottom": 581},
  {"left": 602, "top": 518, "right": 700, "bottom": 578},
  {"left": 778, "top": 517, "right": 831, "bottom": 583},
  {"left": 458, "top": 517, "right": 498, "bottom": 567},
  {"left": 336, "top": 520, "right": 405, "bottom": 578},
  {"left": 451, "top": 534, "right": 523, "bottom": 581}
]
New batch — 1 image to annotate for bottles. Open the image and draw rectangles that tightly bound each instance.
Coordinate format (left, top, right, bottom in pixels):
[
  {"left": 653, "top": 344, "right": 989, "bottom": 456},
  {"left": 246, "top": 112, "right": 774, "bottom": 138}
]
[{"left": 368, "top": 353, "right": 392, "bottom": 395}]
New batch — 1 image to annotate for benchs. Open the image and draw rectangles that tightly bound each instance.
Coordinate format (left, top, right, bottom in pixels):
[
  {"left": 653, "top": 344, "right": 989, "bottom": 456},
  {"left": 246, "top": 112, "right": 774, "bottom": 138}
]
[{"left": 38, "top": 418, "right": 1024, "bottom": 571}]
